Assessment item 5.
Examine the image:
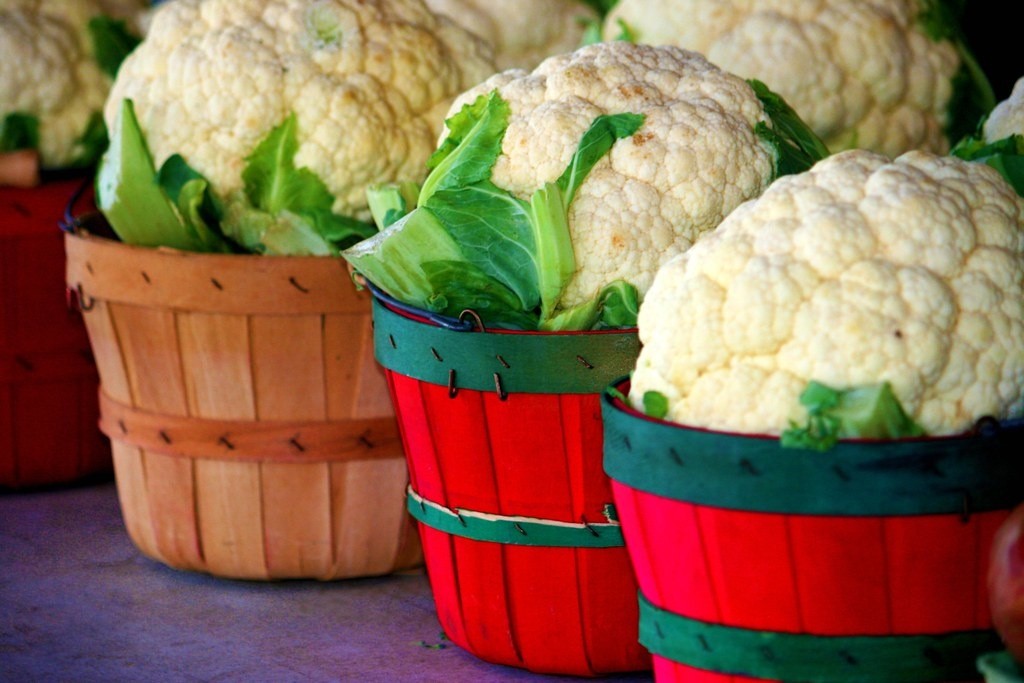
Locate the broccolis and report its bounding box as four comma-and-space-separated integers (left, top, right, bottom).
0, 0, 1023, 447
